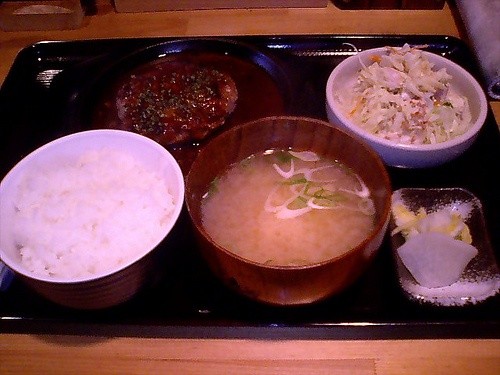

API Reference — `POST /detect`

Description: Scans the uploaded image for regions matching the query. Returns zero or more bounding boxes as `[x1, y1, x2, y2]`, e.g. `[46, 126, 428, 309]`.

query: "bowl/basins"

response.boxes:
[186, 116, 389, 304]
[0, 129, 186, 308]
[326, 48, 489, 171]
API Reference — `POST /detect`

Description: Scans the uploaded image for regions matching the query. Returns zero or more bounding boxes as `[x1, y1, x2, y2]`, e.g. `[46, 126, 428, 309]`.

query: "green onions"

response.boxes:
[263, 150, 376, 219]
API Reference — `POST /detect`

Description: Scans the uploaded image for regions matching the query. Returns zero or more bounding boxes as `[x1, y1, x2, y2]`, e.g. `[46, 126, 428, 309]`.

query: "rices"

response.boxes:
[11, 149, 176, 279]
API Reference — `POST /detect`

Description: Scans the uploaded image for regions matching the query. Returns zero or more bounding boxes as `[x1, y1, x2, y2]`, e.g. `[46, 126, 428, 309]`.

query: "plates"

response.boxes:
[90, 38, 312, 166]
[391, 186, 500, 305]
